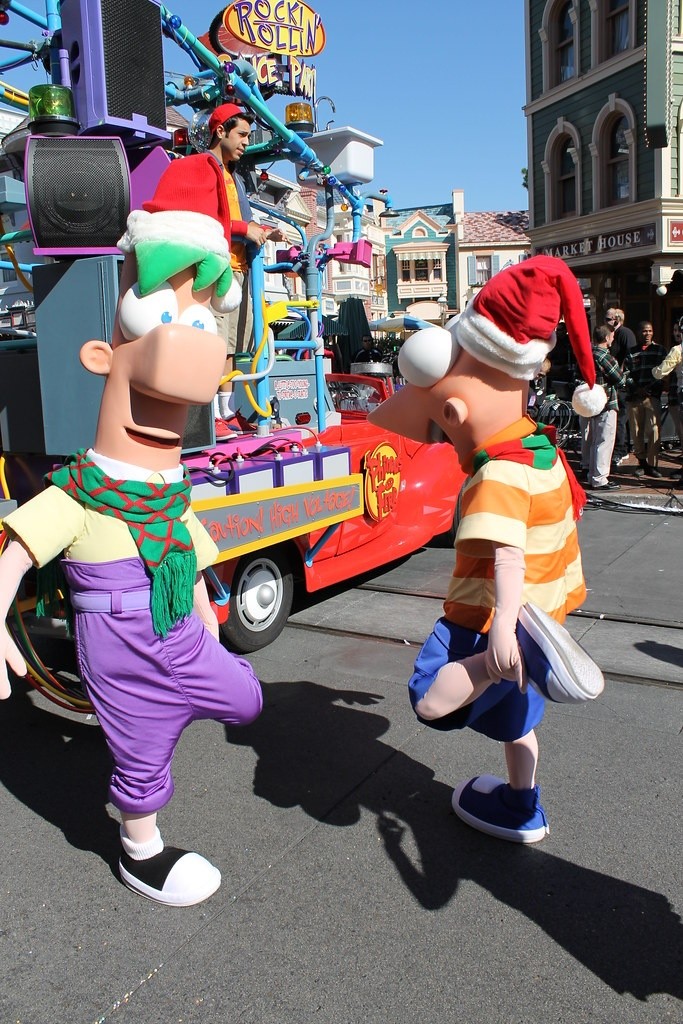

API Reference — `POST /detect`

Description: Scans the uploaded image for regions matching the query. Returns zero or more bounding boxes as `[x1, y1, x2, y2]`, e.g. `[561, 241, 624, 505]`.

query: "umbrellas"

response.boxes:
[368, 314, 442, 339]
[337, 297, 374, 374]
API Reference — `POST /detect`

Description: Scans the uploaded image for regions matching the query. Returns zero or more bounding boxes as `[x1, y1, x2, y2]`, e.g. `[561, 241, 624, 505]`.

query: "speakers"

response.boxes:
[22, 0, 216, 456]
[276, 246, 302, 264]
[334, 240, 373, 268]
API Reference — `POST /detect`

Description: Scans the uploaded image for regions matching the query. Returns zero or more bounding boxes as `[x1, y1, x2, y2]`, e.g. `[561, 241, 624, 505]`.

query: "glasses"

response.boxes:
[605, 318, 617, 322]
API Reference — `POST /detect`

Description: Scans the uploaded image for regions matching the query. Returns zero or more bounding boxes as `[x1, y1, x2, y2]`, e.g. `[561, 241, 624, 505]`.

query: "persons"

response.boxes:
[569, 323, 626, 490]
[354, 335, 383, 363]
[604, 308, 637, 465]
[623, 321, 669, 478]
[546, 322, 576, 365]
[652, 315, 683, 489]
[527, 358, 551, 421]
[204, 104, 292, 442]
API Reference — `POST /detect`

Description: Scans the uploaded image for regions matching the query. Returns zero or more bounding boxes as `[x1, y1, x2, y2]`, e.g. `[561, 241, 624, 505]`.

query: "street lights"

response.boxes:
[437, 292, 448, 327]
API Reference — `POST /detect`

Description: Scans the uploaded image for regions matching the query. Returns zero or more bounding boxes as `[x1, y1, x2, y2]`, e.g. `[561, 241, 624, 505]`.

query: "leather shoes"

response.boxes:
[594, 481, 621, 490]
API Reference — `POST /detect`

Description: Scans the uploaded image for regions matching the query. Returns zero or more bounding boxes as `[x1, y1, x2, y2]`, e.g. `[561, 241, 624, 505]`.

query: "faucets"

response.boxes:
[314, 96, 336, 133]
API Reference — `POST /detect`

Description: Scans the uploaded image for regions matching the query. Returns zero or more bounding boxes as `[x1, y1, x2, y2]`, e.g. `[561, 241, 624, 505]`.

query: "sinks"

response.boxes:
[295, 126, 383, 190]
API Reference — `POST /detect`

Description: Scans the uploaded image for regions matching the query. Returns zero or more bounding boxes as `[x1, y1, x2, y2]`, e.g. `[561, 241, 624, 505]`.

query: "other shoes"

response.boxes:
[612, 457, 622, 465]
[118, 826, 223, 907]
[671, 469, 683, 479]
[515, 602, 604, 705]
[622, 452, 631, 460]
[450, 775, 549, 843]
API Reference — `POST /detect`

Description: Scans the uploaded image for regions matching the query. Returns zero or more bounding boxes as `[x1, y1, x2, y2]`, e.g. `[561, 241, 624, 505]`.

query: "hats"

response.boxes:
[209, 104, 242, 137]
[456, 254, 608, 418]
[115, 154, 232, 258]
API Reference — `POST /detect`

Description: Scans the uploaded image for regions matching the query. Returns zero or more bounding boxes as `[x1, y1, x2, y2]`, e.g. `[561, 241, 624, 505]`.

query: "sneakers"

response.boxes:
[634, 466, 646, 476]
[645, 467, 663, 478]
[225, 408, 258, 435]
[215, 417, 237, 441]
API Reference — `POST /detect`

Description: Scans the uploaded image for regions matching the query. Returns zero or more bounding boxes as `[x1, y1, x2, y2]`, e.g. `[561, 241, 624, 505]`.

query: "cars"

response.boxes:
[0, 371, 471, 651]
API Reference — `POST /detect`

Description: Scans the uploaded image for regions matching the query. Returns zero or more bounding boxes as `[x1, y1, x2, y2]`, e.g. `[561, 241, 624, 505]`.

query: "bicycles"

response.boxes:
[534, 371, 586, 457]
[373, 335, 410, 377]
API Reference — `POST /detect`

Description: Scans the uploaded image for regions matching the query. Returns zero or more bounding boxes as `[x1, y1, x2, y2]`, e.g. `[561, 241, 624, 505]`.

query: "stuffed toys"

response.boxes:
[367, 254, 607, 843]
[0, 153, 263, 906]
